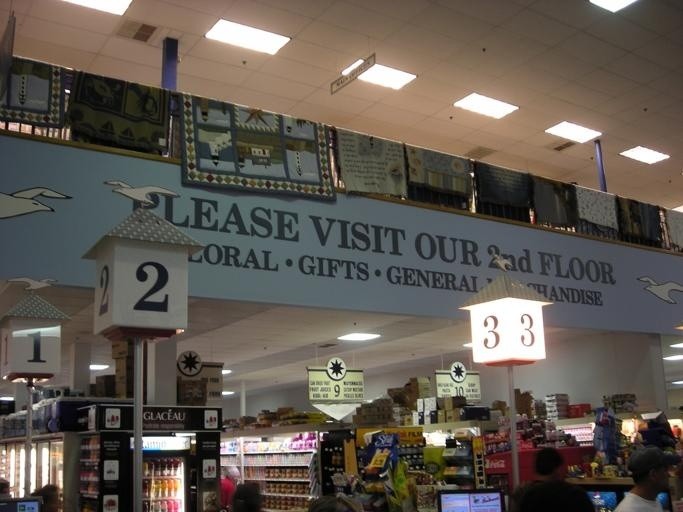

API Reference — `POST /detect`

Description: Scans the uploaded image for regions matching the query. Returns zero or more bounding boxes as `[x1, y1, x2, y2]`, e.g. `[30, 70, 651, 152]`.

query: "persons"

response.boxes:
[231, 482, 267, 511]
[0, 477, 59, 512]
[219, 466, 240, 511]
[613, 447, 682, 512]
[507, 447, 596, 512]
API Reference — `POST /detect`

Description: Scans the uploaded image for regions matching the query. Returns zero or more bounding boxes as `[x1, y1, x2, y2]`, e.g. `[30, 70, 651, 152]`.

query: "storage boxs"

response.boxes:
[514, 387, 591, 420]
[237, 405, 326, 428]
[353, 375, 511, 426]
[89, 338, 133, 398]
[176, 372, 207, 406]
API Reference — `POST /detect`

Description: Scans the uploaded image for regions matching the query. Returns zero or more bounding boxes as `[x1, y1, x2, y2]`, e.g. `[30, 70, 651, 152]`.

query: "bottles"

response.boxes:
[142, 458, 182, 512]
[79, 436, 99, 493]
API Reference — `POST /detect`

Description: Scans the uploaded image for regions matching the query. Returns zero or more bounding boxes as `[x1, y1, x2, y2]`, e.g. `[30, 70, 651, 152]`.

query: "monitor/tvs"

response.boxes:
[624, 491, 673, 512]
[16, 500, 39, 512]
[437, 488, 506, 512]
[586, 491, 617, 512]
[640, 411, 673, 437]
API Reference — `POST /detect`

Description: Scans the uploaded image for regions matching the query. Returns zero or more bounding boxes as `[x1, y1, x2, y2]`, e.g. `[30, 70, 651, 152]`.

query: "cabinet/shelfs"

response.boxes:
[219, 419, 596, 511]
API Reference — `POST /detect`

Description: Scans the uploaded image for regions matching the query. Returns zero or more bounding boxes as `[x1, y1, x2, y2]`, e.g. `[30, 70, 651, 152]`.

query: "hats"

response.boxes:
[627, 444, 682, 472]
